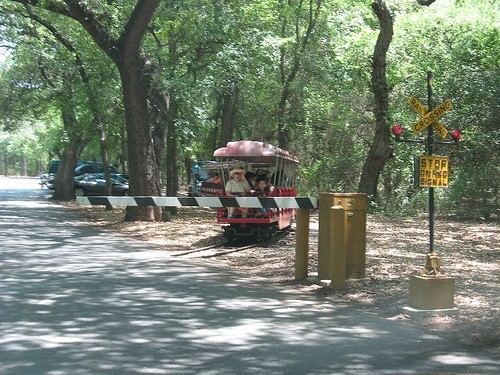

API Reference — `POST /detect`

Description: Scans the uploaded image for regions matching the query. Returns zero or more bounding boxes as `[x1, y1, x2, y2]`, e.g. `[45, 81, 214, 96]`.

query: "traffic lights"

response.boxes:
[391, 125, 402, 136]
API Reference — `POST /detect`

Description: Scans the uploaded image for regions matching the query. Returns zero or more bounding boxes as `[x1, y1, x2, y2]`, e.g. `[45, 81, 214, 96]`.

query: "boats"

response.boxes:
[448, 127, 462, 142]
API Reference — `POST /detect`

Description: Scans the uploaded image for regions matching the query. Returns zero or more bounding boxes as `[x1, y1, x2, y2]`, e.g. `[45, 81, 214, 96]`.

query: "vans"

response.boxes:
[46, 159, 121, 191]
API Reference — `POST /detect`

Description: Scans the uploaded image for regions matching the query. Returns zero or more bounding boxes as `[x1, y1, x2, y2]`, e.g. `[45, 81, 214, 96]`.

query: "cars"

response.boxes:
[69, 172, 129, 195]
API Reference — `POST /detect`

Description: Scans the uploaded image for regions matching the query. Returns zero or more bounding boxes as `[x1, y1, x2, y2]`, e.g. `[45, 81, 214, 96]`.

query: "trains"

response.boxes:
[189, 140, 307, 245]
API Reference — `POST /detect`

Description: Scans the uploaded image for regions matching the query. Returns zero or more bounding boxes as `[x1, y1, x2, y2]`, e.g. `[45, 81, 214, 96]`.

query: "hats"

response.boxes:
[229, 167, 244, 177]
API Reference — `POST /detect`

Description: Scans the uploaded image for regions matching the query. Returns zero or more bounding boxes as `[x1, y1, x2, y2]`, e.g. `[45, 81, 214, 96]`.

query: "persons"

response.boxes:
[224, 168, 252, 219]
[244, 165, 296, 192]
[207, 173, 222, 184]
[251, 177, 271, 219]
[39, 171, 49, 189]
